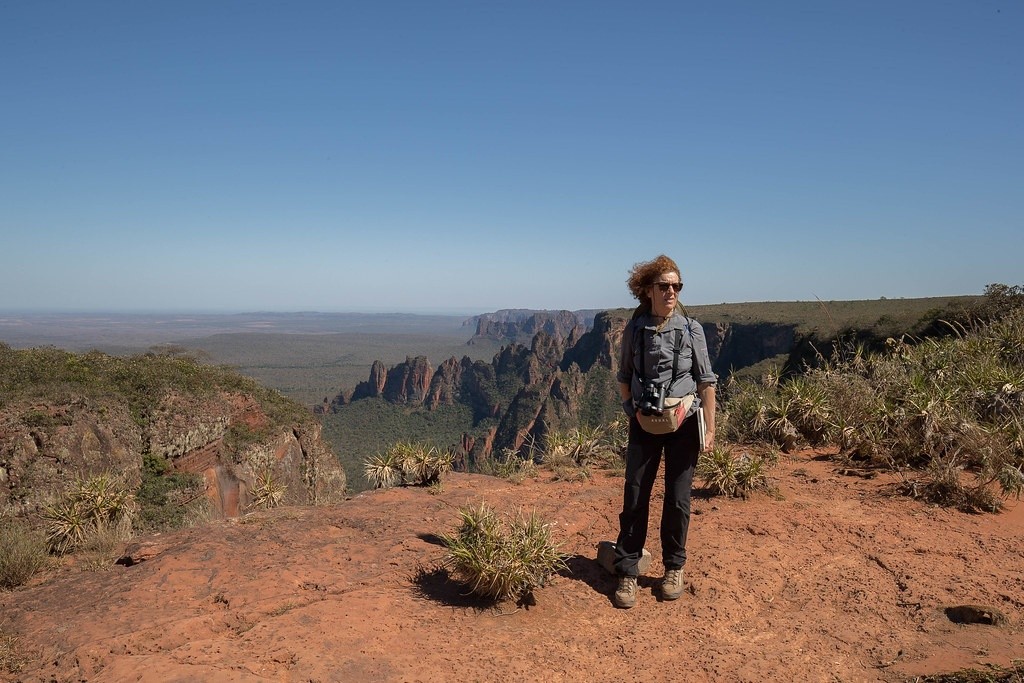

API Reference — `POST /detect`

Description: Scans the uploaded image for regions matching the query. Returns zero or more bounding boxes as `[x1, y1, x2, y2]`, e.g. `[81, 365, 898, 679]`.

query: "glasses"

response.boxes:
[648, 282, 683, 293]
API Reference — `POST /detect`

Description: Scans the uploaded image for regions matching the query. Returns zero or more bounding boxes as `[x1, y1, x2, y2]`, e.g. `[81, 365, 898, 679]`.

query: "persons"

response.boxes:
[614, 254, 717, 608]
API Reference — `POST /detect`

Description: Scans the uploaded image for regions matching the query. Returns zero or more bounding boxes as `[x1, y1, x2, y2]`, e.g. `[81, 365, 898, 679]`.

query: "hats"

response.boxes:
[632, 300, 689, 317]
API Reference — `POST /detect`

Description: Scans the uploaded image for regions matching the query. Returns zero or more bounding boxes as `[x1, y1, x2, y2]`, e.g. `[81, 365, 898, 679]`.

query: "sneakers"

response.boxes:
[661, 568, 684, 598]
[615, 577, 637, 607]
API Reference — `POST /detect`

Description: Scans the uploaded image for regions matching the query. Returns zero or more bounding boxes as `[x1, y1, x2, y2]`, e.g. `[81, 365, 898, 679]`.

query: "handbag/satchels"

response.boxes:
[632, 395, 694, 434]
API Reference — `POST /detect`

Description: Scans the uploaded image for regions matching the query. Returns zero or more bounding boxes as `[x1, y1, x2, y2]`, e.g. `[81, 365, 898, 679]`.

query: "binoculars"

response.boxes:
[640, 381, 667, 417]
